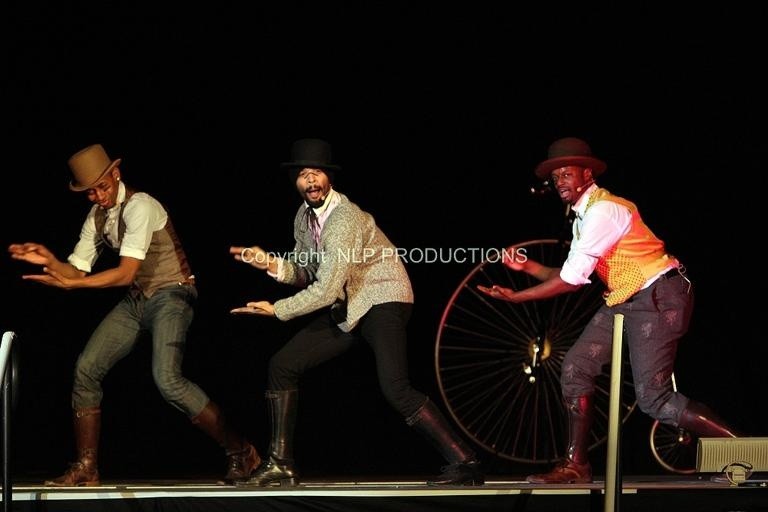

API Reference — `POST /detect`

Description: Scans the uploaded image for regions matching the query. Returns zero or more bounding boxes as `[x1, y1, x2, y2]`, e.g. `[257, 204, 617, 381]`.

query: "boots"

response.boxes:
[187, 396, 262, 486]
[678, 398, 754, 484]
[43, 405, 102, 488]
[526, 389, 595, 485]
[403, 395, 486, 487]
[233, 386, 301, 489]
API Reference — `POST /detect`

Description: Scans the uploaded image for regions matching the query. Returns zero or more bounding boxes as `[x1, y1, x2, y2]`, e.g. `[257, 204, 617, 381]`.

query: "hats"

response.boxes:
[66, 143, 123, 193]
[534, 137, 609, 180]
[280, 138, 343, 172]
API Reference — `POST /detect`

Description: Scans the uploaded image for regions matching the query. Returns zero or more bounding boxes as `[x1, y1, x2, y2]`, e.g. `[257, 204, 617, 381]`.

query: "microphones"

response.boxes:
[99, 206, 104, 210]
[319, 191, 331, 200]
[577, 181, 596, 193]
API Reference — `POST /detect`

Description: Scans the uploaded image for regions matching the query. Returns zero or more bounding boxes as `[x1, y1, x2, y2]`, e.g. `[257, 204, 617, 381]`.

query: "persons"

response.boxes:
[475, 135, 758, 485]
[229, 136, 487, 488]
[6, 141, 265, 487]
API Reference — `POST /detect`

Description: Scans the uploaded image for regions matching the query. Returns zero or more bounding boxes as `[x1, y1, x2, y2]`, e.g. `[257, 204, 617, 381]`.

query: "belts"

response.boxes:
[665, 268, 687, 278]
